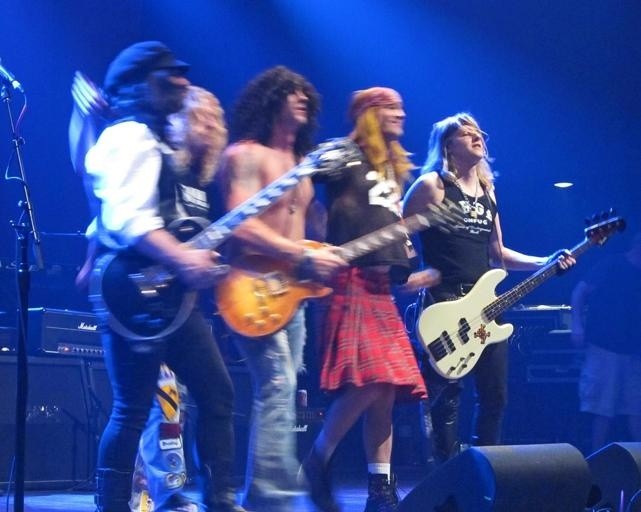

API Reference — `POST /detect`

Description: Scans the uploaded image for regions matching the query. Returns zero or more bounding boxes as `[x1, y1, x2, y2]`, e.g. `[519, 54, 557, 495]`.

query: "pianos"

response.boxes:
[501, 306, 574, 331]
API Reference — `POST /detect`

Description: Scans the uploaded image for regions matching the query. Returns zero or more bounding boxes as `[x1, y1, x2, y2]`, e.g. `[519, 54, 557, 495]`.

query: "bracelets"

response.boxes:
[293, 243, 316, 276]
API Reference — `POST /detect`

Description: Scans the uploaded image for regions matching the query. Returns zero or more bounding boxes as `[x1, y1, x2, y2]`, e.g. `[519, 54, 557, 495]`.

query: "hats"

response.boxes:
[104, 40, 192, 91]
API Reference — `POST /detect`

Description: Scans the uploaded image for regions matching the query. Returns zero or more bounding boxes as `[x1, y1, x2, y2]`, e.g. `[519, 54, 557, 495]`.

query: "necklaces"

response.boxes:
[276, 153, 302, 218]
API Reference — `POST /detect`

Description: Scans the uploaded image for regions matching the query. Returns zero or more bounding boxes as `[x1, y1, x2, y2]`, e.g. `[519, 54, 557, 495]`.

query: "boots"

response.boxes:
[363, 471, 402, 512]
[299, 446, 338, 511]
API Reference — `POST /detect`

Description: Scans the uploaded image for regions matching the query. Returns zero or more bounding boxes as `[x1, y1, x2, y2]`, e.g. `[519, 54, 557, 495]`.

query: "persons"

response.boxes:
[400, 110, 577, 476]
[65, 70, 231, 511]
[565, 233, 641, 455]
[78, 39, 249, 509]
[292, 83, 449, 511]
[214, 66, 356, 508]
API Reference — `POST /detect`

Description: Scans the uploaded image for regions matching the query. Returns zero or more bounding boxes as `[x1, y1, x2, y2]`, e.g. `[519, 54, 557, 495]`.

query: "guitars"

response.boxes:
[90, 139, 361, 338]
[214, 200, 464, 338]
[415, 208, 627, 380]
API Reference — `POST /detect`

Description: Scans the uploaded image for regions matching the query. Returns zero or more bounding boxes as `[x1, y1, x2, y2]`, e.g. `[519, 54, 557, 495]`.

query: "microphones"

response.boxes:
[0, 65, 25, 94]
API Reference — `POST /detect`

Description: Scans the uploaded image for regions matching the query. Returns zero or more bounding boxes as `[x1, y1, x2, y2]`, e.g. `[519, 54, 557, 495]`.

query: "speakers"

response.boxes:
[584, 442, 641, 512]
[393, 443, 593, 512]
[0, 355, 93, 490]
[86, 360, 114, 490]
